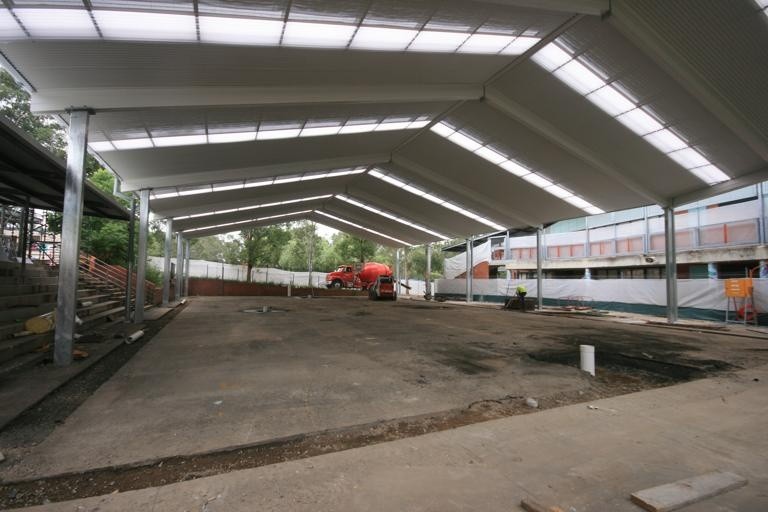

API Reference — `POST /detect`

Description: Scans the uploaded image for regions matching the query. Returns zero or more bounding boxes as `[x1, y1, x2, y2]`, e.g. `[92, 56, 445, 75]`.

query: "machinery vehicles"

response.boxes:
[325, 262, 393, 291]
[368, 274, 396, 301]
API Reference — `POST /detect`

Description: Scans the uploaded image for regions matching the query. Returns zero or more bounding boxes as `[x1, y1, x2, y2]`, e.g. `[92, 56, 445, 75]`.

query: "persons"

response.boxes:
[516, 286, 527, 313]
